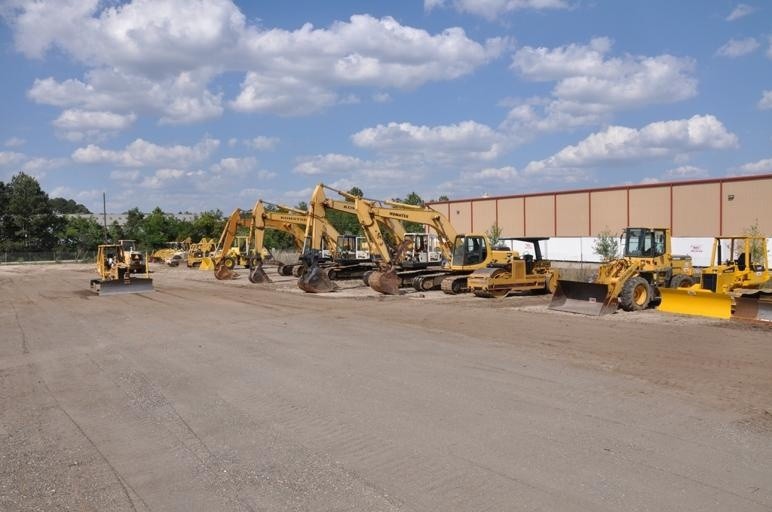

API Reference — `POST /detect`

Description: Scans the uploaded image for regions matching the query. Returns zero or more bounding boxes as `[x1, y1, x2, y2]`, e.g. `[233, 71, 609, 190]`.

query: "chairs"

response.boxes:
[737, 253, 751, 270]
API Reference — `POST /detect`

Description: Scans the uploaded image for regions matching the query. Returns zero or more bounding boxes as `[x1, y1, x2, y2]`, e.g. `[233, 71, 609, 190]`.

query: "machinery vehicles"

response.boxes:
[87, 239, 155, 297]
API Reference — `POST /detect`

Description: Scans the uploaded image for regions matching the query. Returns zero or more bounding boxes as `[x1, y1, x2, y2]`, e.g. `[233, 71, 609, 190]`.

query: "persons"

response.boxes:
[211, 244, 216, 251]
[646, 242, 657, 254]
